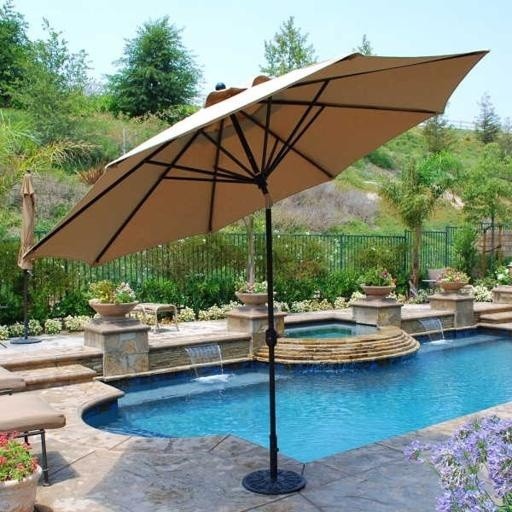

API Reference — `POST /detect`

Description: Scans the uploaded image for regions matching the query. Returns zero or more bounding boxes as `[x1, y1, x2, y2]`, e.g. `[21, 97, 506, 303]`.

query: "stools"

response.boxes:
[1, 394, 66, 486]
[0, 364, 29, 393]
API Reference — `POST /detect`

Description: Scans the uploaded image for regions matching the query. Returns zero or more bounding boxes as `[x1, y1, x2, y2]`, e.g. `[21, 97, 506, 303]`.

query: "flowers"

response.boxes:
[356, 265, 398, 286]
[87, 278, 136, 305]
[435, 268, 469, 282]
[0, 429, 40, 482]
[233, 280, 271, 294]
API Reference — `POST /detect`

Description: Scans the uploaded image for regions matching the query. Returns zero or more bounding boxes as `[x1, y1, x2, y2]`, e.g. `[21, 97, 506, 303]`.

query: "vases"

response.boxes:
[360, 284, 396, 298]
[88, 299, 139, 317]
[0, 464, 43, 512]
[235, 291, 276, 306]
[437, 283, 467, 293]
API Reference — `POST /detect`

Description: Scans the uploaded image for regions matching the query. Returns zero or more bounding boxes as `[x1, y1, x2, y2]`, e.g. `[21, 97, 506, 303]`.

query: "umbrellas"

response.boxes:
[18, 48, 492, 483]
[17, 165, 40, 339]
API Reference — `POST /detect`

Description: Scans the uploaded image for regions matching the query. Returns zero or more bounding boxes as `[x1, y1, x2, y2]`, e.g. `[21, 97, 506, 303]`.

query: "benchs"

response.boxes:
[127, 303, 181, 334]
